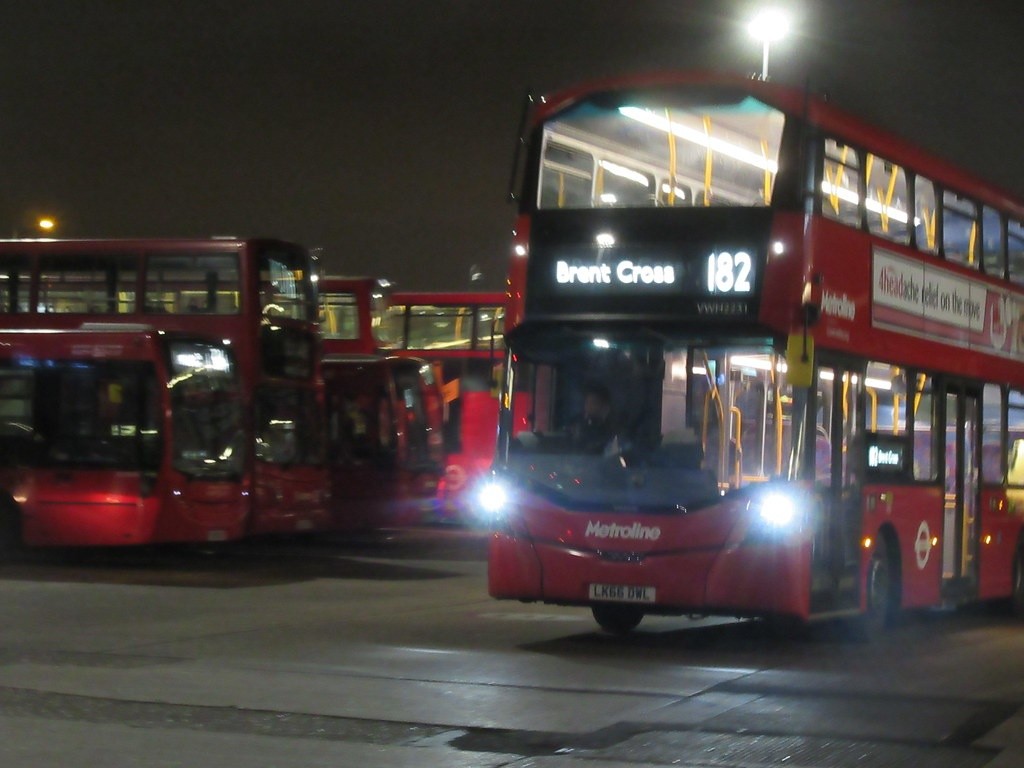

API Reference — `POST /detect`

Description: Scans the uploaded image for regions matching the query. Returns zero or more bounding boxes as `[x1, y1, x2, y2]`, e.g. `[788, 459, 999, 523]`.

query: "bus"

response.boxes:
[349, 288, 508, 551]
[480, 69, 1024, 637]
[0, 321, 256, 573]
[0, 234, 328, 542]
[232, 275, 447, 545]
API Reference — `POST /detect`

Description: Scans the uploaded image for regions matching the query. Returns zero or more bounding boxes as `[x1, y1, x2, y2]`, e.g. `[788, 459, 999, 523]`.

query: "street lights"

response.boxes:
[732, 4, 793, 81]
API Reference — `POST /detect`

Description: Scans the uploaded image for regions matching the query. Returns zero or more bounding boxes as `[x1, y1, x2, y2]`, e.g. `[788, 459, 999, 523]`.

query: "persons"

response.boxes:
[566, 382, 632, 456]
